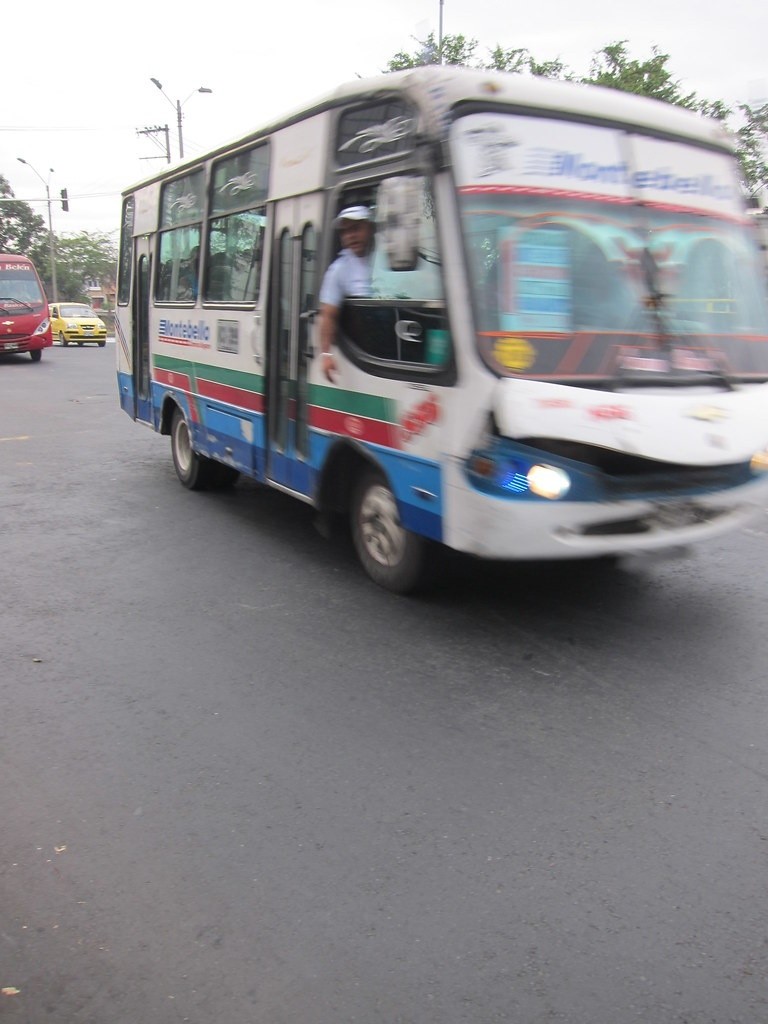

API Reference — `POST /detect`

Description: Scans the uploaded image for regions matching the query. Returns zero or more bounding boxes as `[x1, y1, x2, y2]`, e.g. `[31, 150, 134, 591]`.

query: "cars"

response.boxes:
[47, 301, 107, 347]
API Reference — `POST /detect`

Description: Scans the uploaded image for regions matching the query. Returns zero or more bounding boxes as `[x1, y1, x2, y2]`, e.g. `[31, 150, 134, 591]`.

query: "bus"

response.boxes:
[0, 252, 53, 362]
[112, 62, 768, 596]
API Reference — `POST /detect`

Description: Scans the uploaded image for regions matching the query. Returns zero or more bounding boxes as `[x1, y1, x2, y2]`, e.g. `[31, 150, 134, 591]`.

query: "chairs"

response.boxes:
[151, 246, 261, 299]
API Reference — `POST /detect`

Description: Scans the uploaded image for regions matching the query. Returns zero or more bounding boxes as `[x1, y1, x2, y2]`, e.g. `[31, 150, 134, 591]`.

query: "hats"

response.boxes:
[331, 205, 372, 228]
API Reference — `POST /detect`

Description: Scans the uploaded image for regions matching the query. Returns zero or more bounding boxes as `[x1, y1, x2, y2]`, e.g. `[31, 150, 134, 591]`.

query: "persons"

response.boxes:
[8, 282, 36, 302]
[318, 206, 405, 384]
[179, 246, 211, 299]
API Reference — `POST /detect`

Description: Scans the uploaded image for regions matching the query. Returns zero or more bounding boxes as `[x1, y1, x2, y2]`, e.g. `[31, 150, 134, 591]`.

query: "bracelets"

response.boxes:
[320, 353, 333, 357]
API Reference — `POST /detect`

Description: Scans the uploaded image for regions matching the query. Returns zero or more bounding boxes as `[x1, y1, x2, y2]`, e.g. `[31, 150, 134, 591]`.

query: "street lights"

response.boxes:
[16, 156, 59, 303]
[150, 77, 212, 159]
